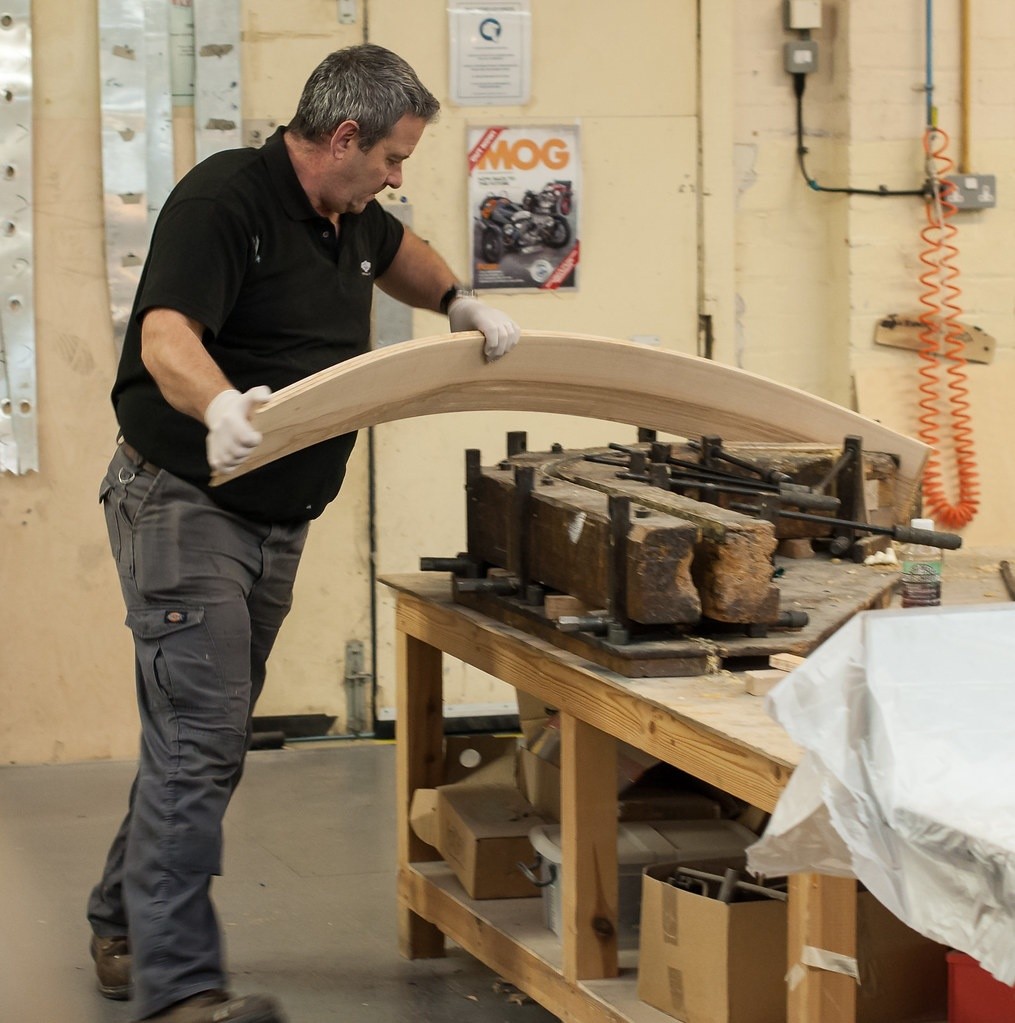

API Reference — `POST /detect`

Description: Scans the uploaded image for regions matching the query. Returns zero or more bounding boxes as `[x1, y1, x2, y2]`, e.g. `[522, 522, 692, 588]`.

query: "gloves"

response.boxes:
[201, 386, 270, 477]
[448, 296, 520, 361]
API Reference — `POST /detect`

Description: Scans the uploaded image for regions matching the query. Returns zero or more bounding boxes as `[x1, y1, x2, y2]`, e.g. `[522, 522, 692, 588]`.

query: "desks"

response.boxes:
[366, 566, 955, 1023]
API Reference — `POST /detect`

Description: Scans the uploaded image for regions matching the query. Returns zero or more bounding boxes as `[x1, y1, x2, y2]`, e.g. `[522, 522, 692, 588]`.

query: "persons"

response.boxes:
[86, 42, 522, 1023]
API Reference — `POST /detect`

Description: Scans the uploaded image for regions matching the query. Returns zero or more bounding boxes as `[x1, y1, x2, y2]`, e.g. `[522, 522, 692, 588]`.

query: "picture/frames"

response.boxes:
[466, 121, 582, 296]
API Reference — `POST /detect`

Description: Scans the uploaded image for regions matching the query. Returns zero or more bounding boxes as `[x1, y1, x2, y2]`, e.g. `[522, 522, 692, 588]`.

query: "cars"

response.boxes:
[479, 181, 574, 264]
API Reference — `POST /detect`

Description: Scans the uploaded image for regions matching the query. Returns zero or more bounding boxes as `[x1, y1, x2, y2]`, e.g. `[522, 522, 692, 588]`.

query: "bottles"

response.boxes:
[902, 517, 942, 607]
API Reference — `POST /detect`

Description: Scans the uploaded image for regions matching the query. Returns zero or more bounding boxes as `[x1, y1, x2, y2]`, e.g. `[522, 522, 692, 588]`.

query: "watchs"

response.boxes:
[440, 282, 478, 315]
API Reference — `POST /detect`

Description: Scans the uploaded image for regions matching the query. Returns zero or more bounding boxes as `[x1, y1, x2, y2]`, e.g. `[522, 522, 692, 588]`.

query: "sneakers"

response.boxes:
[141, 992, 287, 1023]
[86, 931, 134, 999]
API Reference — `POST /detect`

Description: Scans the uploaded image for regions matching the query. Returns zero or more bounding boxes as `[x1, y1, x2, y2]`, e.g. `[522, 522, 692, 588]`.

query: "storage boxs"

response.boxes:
[782, 864, 949, 1022]
[414, 730, 720, 900]
[945, 950, 1015, 1023]
[634, 857, 788, 1023]
